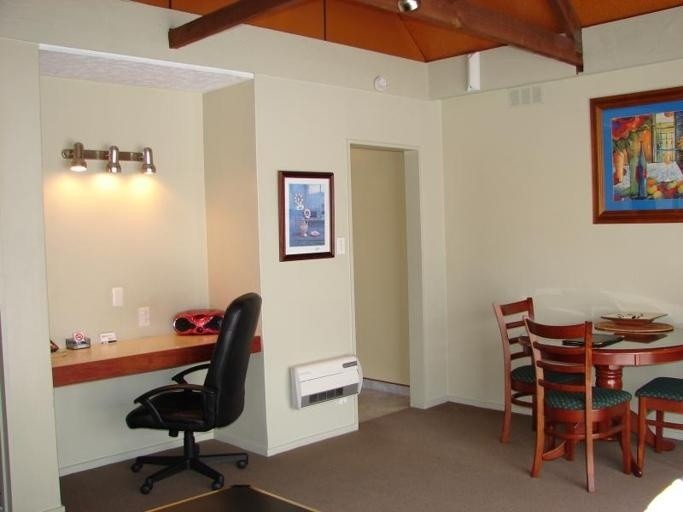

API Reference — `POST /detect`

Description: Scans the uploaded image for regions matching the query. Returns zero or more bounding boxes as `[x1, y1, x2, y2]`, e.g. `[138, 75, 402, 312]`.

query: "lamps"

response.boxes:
[61, 142, 156, 176]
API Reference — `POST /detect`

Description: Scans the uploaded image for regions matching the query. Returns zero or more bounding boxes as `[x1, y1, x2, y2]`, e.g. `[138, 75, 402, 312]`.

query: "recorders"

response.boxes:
[172, 308, 224, 336]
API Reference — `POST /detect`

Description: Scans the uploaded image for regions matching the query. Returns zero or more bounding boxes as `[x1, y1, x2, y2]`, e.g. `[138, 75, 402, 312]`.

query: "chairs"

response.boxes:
[492, 297, 682, 493]
[125, 292, 262, 495]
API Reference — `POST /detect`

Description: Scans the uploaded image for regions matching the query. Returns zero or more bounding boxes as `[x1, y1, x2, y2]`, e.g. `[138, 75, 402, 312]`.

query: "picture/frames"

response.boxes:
[277, 170, 335, 262]
[589, 85, 683, 223]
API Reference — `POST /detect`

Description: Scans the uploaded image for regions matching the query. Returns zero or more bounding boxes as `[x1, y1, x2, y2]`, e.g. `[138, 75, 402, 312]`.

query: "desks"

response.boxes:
[51, 332, 262, 388]
